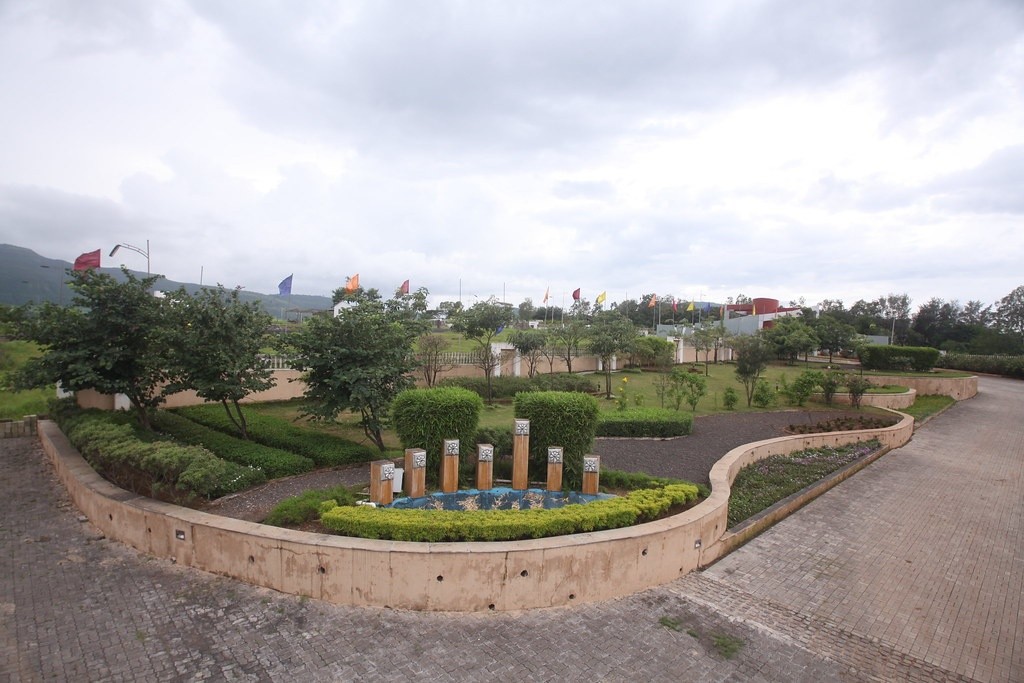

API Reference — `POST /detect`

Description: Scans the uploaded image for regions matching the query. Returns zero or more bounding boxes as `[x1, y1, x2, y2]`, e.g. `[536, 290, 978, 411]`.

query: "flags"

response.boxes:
[598, 292, 606, 303]
[673, 303, 677, 311]
[278, 274, 292, 297]
[400, 280, 409, 293]
[346, 274, 359, 295]
[705, 303, 712, 312]
[543, 287, 549, 302]
[74, 249, 100, 272]
[648, 294, 656, 307]
[687, 300, 695, 311]
[573, 288, 580, 299]
